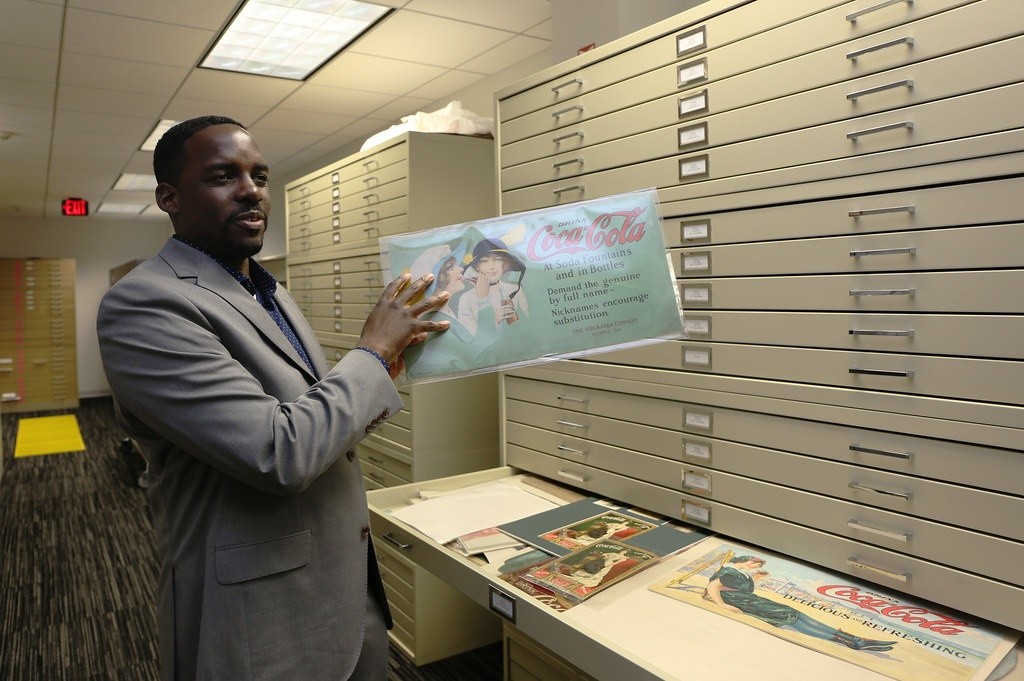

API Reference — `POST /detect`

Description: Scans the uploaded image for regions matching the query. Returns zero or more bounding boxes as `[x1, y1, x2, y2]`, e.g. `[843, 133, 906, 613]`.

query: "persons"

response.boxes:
[96, 116, 450, 681]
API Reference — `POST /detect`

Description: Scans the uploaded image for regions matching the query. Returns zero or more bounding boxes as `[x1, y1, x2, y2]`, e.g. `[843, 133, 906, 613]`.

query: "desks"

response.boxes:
[365, 464, 1024, 681]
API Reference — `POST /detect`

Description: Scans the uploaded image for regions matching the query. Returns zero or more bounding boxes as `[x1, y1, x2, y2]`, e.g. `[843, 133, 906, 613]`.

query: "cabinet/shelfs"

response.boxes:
[0, 257, 79, 412]
[370, 534, 501, 666]
[503, 618, 598, 680]
[283, 128, 500, 492]
[494, 0, 1023, 636]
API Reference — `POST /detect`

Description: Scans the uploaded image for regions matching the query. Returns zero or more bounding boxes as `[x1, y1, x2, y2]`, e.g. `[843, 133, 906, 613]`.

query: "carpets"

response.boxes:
[14, 413, 85, 457]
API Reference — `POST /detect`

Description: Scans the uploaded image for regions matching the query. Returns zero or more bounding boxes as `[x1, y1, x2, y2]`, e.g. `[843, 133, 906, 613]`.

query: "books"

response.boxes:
[409, 480, 659, 613]
